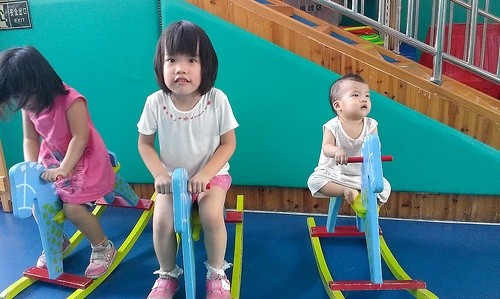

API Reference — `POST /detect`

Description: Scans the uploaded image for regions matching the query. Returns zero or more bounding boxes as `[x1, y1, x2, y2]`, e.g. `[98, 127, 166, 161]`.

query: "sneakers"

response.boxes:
[85, 239, 116, 278]
[36, 234, 72, 269]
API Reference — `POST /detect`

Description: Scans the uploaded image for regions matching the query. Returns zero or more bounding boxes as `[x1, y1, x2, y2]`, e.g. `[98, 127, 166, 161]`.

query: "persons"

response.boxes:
[307, 72, 392, 209]
[136, 19, 240, 299]
[0, 45, 117, 280]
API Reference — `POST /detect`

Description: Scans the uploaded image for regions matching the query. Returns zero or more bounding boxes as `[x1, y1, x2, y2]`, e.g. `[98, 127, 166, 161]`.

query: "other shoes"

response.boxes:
[147, 276, 179, 299]
[206, 273, 231, 299]
